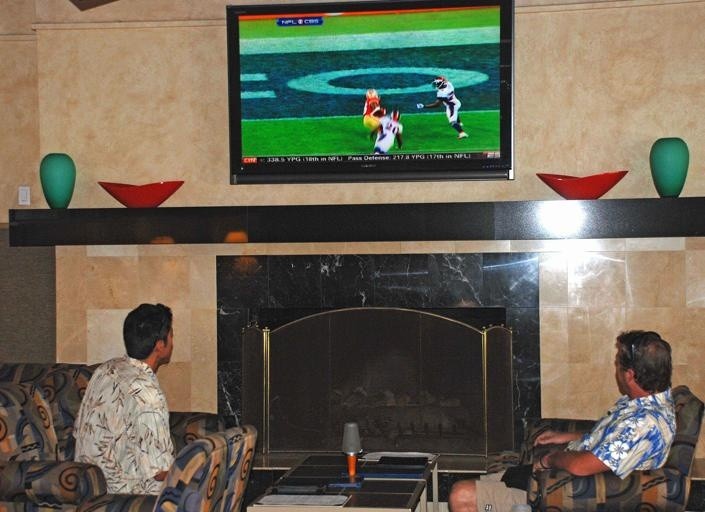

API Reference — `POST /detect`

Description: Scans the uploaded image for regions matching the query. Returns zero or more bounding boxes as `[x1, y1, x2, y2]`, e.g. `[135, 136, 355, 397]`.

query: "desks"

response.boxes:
[243, 452, 439, 512]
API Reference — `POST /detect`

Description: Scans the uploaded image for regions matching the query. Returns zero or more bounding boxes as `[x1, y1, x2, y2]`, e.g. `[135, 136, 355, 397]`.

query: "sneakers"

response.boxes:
[460, 123, 464, 130]
[458, 132, 468, 139]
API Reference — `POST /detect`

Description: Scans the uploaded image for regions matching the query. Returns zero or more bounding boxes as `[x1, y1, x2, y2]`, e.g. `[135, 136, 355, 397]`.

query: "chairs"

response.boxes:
[1, 361, 258, 512]
[487, 386, 705, 512]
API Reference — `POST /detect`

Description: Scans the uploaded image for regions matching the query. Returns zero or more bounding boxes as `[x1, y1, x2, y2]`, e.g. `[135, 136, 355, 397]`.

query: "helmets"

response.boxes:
[390, 109, 401, 121]
[365, 88, 378, 99]
[432, 77, 448, 89]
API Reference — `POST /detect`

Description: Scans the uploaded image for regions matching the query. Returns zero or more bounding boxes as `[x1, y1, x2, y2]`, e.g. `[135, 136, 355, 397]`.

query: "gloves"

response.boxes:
[417, 103, 424, 109]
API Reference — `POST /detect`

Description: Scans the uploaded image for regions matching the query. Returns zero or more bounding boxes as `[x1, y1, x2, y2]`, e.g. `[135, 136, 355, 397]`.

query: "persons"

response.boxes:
[448, 331, 677, 512]
[72, 303, 175, 495]
[363, 89, 403, 155]
[417, 77, 469, 139]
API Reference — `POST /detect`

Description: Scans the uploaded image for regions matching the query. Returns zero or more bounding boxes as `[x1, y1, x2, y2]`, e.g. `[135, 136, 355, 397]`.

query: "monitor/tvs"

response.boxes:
[226, 0, 515, 185]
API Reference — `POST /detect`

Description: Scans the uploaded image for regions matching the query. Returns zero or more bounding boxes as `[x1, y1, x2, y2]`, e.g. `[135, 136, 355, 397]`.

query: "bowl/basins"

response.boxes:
[96, 181, 187, 208]
[535, 171, 629, 200]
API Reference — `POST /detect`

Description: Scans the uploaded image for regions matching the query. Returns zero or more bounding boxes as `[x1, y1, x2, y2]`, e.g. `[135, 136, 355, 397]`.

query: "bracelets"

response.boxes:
[540, 453, 552, 469]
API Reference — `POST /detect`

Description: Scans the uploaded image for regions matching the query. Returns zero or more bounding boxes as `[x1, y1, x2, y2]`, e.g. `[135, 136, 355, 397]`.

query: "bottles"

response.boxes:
[39, 153, 76, 209]
[650, 138, 689, 198]
